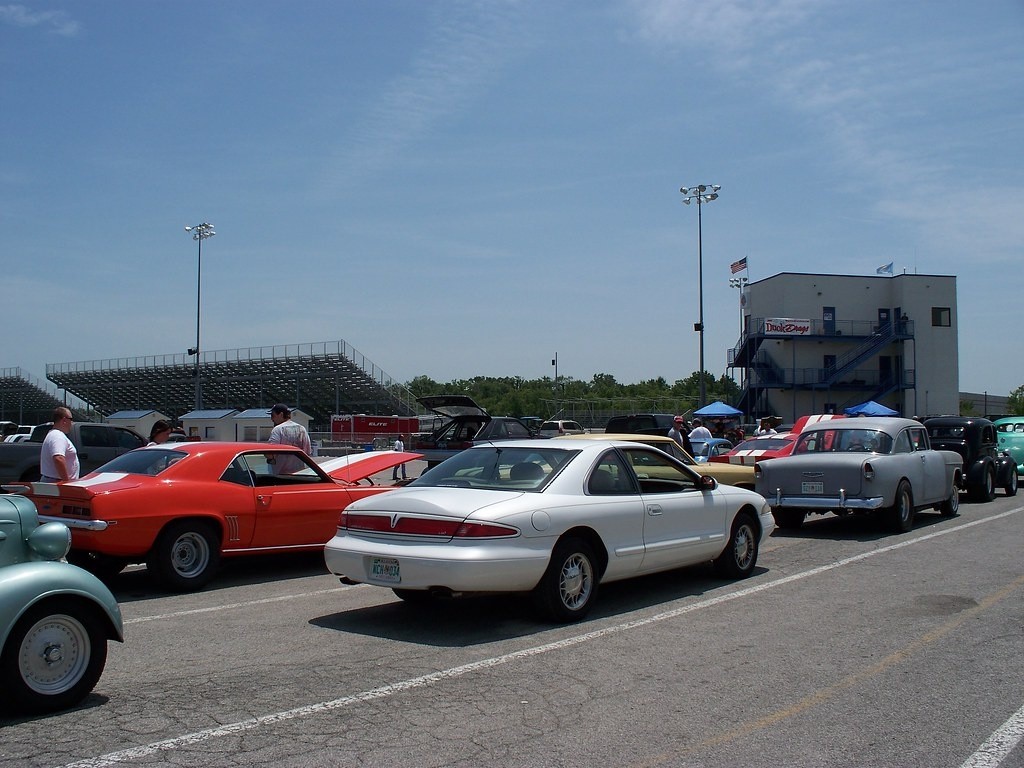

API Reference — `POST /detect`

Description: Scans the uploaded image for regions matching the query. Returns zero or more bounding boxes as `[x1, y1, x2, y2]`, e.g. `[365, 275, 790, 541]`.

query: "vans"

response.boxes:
[605, 414, 694, 456]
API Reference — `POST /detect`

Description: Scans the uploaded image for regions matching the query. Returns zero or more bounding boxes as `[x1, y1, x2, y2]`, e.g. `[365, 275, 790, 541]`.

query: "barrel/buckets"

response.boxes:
[836, 331, 841, 336]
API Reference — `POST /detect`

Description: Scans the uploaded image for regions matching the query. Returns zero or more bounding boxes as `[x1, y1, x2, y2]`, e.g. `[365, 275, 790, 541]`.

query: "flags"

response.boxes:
[730, 256, 747, 274]
[876, 261, 893, 274]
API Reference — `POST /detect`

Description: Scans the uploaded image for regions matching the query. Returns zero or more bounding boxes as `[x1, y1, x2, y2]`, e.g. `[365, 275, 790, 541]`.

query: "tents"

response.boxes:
[841, 401, 899, 417]
[692, 401, 745, 427]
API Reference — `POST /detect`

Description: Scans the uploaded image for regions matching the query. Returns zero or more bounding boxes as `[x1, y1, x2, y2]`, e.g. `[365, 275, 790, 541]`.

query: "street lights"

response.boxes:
[681, 182, 724, 409]
[185, 221, 216, 410]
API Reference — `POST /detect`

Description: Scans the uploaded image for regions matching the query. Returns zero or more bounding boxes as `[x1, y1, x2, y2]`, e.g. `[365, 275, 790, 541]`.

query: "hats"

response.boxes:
[673, 415, 683, 423]
[266, 403, 291, 414]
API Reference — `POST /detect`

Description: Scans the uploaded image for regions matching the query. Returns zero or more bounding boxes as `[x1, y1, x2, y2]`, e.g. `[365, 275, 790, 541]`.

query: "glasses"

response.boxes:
[61, 417, 74, 421]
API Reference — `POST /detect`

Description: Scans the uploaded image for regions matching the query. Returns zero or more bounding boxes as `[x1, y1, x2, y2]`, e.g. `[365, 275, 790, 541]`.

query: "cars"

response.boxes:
[0, 420, 35, 444]
[411, 393, 538, 469]
[25, 441, 425, 596]
[705, 412, 847, 467]
[454, 431, 758, 494]
[755, 416, 967, 532]
[688, 436, 734, 464]
[988, 416, 1024, 477]
[535, 419, 586, 438]
[914, 415, 1018, 502]
[0, 492, 128, 716]
[324, 437, 777, 625]
[772, 424, 793, 433]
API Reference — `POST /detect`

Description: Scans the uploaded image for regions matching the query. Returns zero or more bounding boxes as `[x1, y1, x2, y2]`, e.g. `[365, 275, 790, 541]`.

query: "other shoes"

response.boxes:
[402, 477, 410, 480]
[393, 478, 401, 480]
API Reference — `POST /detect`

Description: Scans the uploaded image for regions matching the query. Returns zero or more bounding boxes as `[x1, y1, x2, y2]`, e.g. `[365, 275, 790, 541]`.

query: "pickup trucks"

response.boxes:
[0, 423, 150, 495]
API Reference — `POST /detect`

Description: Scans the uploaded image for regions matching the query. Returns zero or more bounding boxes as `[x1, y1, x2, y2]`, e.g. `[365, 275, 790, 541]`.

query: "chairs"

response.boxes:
[509, 462, 545, 480]
[589, 469, 620, 491]
[468, 425, 477, 437]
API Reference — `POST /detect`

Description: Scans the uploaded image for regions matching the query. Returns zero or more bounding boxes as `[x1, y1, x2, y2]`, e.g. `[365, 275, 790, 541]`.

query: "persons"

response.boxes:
[753, 422, 777, 437]
[910, 415, 920, 442]
[668, 416, 744, 460]
[39, 407, 79, 483]
[847, 414, 875, 450]
[392, 434, 410, 481]
[262, 404, 312, 476]
[146, 421, 186, 447]
